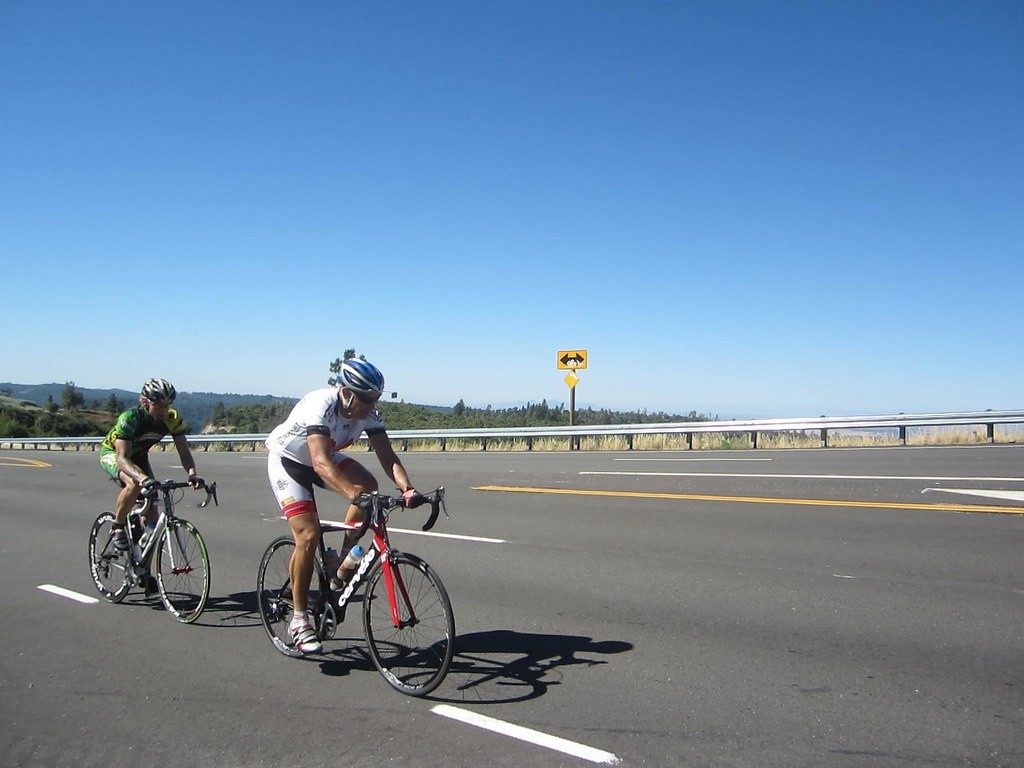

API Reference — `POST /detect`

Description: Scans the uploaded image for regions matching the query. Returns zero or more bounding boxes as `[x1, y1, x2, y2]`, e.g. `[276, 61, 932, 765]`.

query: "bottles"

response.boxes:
[326, 547, 342, 591]
[138, 521, 156, 549]
[336, 545, 366, 582]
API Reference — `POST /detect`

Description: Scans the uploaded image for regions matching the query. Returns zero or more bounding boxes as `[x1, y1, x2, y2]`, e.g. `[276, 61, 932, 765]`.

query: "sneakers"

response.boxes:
[337, 564, 370, 581]
[288, 620, 323, 654]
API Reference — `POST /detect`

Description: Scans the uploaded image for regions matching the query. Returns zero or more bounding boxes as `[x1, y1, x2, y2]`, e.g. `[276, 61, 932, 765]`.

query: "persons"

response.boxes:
[266, 357, 422, 654]
[101, 378, 205, 586]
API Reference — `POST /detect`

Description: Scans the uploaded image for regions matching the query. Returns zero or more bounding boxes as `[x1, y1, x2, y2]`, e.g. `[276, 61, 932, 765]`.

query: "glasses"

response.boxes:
[147, 399, 173, 409]
[348, 388, 381, 404]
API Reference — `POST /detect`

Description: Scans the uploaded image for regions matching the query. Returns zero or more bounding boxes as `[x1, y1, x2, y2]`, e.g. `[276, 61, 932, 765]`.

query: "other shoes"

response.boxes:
[109, 526, 129, 551]
[139, 576, 159, 593]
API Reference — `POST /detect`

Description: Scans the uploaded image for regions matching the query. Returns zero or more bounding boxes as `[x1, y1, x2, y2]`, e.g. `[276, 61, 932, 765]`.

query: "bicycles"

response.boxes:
[88, 477, 220, 625]
[256, 483, 457, 697]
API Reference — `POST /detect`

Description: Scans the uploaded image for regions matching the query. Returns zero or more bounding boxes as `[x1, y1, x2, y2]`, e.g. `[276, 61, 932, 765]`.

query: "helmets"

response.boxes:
[340, 358, 384, 394]
[141, 378, 176, 404]
